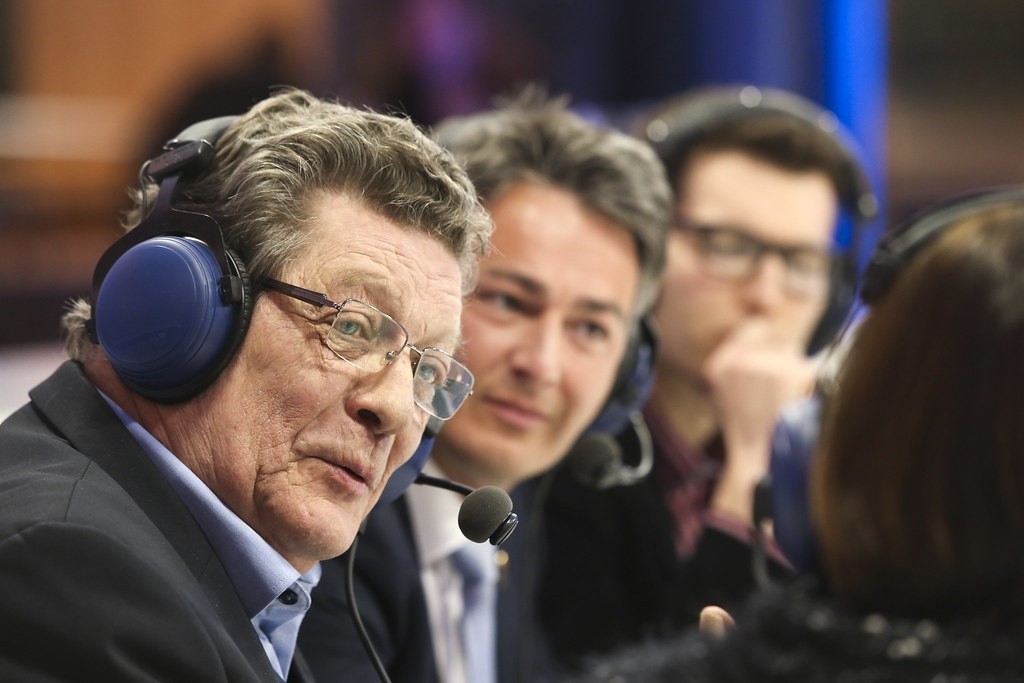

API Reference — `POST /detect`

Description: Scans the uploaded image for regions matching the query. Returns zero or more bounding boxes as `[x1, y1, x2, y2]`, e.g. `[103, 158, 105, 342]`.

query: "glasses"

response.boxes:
[256, 275, 475, 421]
[673, 225, 846, 290]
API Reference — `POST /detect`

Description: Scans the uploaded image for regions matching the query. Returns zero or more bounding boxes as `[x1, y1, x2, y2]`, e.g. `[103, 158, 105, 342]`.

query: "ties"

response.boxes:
[448, 536, 501, 683]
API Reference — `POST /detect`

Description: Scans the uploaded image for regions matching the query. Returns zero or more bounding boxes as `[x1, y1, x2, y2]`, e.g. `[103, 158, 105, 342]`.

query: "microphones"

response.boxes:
[414, 472, 519, 546]
[581, 410, 653, 488]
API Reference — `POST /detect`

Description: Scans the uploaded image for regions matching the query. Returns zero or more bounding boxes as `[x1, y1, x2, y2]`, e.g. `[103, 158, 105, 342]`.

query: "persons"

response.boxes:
[575, 86, 876, 609]
[0, 91, 492, 683]
[347, 104, 676, 683]
[609, 203, 1024, 683]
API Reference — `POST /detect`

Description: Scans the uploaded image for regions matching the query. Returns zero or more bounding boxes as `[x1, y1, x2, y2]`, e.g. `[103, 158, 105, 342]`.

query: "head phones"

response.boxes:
[86, 116, 443, 505]
[564, 77, 1024, 577]
